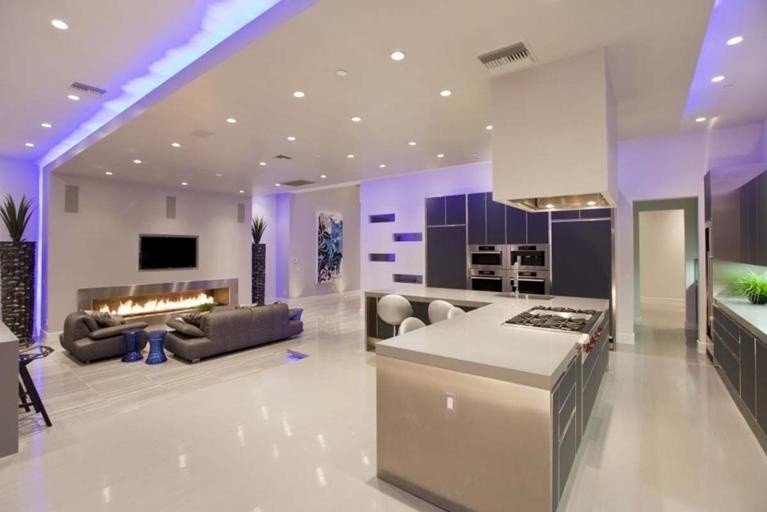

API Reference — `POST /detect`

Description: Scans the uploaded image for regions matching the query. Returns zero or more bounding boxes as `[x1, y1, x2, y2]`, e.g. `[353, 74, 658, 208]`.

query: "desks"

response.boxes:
[0, 318, 22, 458]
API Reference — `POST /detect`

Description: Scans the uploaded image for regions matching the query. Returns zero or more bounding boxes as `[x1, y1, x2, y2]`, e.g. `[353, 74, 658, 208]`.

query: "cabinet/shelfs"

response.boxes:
[738, 170, 767, 268]
[712, 303, 767, 456]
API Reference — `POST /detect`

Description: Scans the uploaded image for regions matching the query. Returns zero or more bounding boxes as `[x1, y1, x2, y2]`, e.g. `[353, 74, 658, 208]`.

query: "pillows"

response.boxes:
[181, 314, 202, 328]
[272, 300, 297, 320]
[84, 309, 120, 326]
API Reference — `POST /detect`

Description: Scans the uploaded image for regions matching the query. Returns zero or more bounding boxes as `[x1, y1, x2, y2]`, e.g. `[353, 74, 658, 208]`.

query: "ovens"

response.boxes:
[466, 243, 552, 295]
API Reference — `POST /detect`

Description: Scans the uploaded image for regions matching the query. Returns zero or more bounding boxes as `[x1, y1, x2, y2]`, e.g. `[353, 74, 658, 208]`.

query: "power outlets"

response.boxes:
[445, 392, 456, 415]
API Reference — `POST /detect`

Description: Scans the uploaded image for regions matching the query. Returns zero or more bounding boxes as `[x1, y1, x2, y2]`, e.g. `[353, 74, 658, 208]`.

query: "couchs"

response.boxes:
[163, 303, 304, 363]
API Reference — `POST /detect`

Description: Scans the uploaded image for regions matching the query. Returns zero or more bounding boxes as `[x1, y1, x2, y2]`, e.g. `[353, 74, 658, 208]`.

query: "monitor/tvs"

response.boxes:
[138, 233, 198, 269]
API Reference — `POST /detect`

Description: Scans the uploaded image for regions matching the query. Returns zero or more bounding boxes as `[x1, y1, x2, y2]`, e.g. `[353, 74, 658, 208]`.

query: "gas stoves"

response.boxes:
[500, 304, 606, 353]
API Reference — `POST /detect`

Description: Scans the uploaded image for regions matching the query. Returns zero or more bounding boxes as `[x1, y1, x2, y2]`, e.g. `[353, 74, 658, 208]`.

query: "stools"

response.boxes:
[17, 337, 55, 426]
[145, 330, 168, 365]
[120, 329, 144, 362]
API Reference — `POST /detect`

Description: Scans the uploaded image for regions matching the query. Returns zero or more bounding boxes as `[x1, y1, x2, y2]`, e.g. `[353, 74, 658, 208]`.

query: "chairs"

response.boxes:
[59, 312, 149, 365]
[376, 292, 465, 338]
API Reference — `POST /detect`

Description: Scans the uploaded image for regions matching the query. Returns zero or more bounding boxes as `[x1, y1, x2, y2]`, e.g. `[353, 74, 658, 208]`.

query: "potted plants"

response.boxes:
[0, 189, 38, 344]
[196, 302, 215, 314]
[729, 269, 767, 305]
[251, 216, 268, 306]
